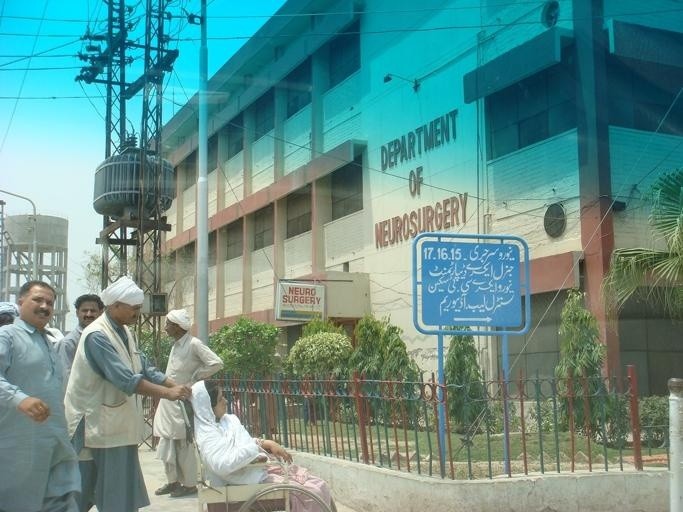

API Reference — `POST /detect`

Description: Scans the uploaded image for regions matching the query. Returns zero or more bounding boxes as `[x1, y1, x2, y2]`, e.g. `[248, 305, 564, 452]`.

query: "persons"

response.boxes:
[186, 378, 337, 512]
[53, 293, 105, 512]
[151, 308, 225, 498]
[63, 274, 194, 512]
[0, 281, 81, 512]
[0, 300, 18, 325]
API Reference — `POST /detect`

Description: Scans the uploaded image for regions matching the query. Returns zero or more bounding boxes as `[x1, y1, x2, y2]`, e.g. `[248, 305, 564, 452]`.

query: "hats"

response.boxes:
[0, 301, 20, 319]
[99, 276, 144, 307]
[166, 308, 191, 331]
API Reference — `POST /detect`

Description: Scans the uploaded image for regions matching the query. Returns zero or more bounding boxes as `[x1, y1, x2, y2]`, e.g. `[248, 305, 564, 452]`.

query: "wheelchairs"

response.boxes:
[174, 397, 331, 512]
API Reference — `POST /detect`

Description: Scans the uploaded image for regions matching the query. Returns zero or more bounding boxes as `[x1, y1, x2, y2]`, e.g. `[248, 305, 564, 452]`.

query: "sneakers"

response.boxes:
[168, 486, 198, 497]
[154, 482, 180, 495]
